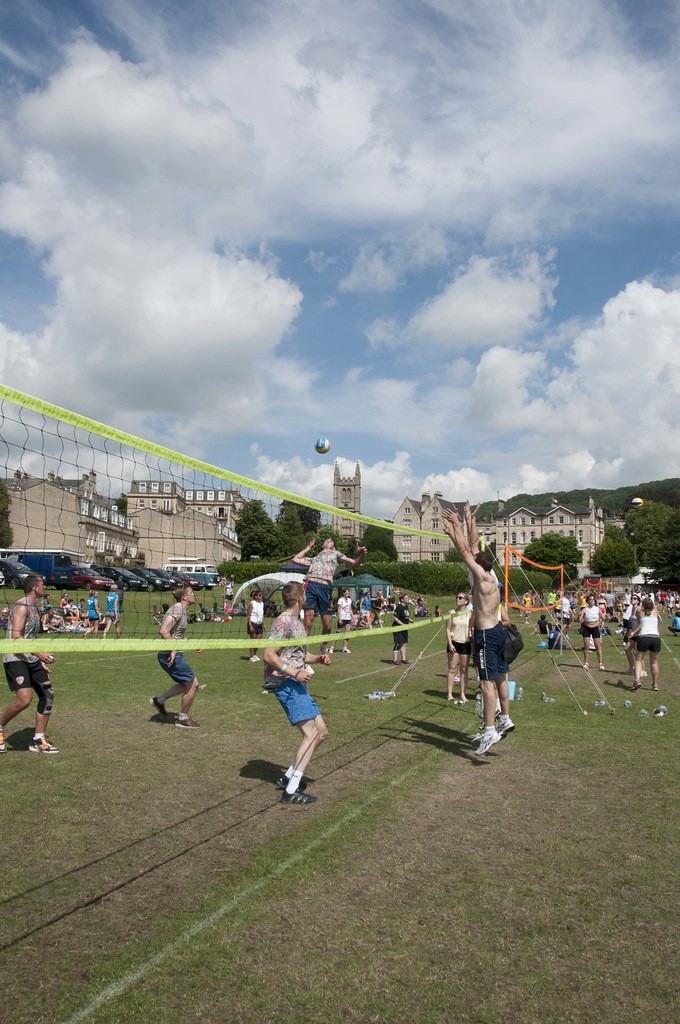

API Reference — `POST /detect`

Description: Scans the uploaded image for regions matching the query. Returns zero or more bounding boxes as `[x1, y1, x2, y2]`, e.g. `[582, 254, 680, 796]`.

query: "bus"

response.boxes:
[162, 563, 221, 590]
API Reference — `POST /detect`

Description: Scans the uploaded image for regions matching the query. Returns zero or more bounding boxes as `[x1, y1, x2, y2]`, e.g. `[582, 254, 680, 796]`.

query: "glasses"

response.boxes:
[631, 597, 638, 600]
[456, 596, 467, 600]
[566, 593, 569, 595]
[256, 594, 262, 596]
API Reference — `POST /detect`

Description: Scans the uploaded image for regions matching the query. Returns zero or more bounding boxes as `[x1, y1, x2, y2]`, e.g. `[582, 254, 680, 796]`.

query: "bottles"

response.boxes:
[475, 692, 482, 717]
[615, 626, 627, 636]
[544, 698, 554, 702]
[517, 685, 523, 700]
[363, 690, 396, 699]
[594, 699, 604, 707]
[659, 704, 667, 714]
[625, 699, 632, 709]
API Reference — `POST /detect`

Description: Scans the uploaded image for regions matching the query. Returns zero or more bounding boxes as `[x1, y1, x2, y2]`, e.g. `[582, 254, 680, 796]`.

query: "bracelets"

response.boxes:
[280, 663, 287, 673]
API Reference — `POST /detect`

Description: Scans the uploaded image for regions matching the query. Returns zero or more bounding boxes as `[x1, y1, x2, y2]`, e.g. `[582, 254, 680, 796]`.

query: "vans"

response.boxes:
[6, 553, 74, 590]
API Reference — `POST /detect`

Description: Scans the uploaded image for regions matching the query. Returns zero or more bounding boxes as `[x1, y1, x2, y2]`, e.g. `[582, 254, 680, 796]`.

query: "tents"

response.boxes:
[331, 574, 393, 600]
[232, 572, 306, 609]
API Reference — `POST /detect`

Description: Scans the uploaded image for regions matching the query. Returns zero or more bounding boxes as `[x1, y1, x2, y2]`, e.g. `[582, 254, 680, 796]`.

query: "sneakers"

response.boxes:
[0, 725, 7, 752]
[321, 644, 329, 656]
[474, 731, 502, 755]
[280, 788, 317, 804]
[29, 734, 60, 753]
[150, 697, 167, 716]
[175, 717, 200, 728]
[277, 778, 307, 791]
[496, 717, 515, 741]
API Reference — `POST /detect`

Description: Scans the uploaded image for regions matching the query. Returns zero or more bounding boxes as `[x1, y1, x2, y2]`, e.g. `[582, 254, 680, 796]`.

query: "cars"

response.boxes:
[93, 566, 148, 591]
[70, 565, 115, 591]
[0, 571, 6, 589]
[0, 559, 47, 589]
[166, 570, 201, 590]
[145, 569, 185, 589]
[127, 568, 172, 591]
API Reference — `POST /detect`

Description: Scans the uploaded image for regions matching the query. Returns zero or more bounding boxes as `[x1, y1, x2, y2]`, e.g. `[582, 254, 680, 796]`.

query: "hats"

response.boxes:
[564, 590, 572, 594]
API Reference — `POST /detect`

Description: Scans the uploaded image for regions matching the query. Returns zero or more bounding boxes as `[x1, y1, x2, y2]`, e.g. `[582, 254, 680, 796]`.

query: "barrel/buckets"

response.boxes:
[506, 681, 516, 700]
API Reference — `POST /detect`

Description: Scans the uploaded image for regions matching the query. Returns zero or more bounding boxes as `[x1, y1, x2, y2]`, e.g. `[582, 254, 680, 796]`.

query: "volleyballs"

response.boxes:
[315, 438, 330, 454]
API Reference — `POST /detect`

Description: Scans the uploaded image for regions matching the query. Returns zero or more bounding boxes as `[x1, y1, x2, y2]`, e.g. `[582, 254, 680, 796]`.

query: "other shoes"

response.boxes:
[344, 648, 351, 654]
[623, 642, 626, 646]
[329, 646, 334, 654]
[600, 662, 604, 670]
[641, 671, 647, 677]
[252, 654, 260, 661]
[630, 680, 641, 691]
[583, 663, 589, 668]
[250, 656, 258, 662]
[653, 684, 658, 692]
[402, 660, 410, 664]
[625, 668, 636, 675]
[393, 660, 401, 665]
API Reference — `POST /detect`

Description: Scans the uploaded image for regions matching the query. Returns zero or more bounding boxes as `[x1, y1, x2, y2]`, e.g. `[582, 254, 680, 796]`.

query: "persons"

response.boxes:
[149, 585, 200, 728]
[238, 599, 246, 612]
[615, 586, 680, 691]
[385, 592, 414, 664]
[408, 595, 440, 617]
[247, 590, 267, 662]
[291, 538, 367, 666]
[439, 499, 615, 756]
[265, 601, 278, 618]
[224, 579, 234, 610]
[0, 575, 59, 753]
[115, 576, 125, 613]
[261, 580, 331, 804]
[82, 590, 102, 639]
[40, 592, 88, 633]
[329, 588, 387, 654]
[102, 583, 121, 639]
